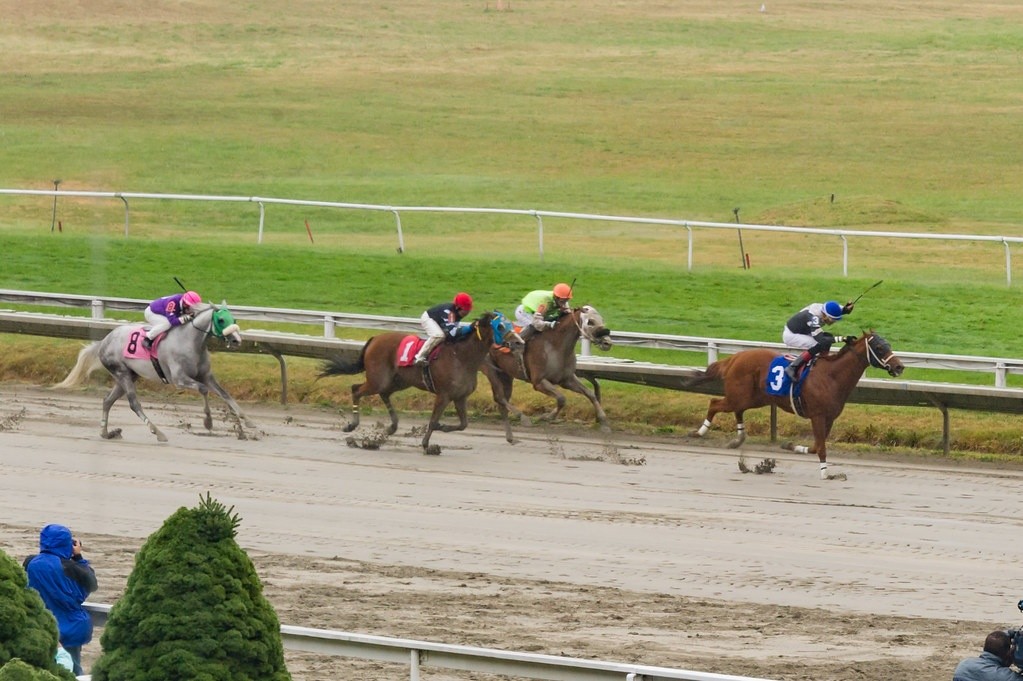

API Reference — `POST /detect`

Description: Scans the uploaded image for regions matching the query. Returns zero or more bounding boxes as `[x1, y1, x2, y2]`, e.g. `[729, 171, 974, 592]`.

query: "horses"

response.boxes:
[46, 299, 257, 441]
[312, 308, 525, 450]
[679, 327, 905, 480]
[476, 305, 613, 445]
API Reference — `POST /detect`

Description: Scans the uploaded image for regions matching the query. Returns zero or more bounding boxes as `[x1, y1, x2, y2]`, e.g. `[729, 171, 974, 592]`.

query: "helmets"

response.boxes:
[455, 293, 472, 310]
[823, 302, 842, 321]
[183, 291, 202, 307]
[555, 283, 573, 301]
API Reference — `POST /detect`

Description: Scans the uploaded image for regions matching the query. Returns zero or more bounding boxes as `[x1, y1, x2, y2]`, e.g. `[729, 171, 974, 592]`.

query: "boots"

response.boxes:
[786, 358, 802, 384]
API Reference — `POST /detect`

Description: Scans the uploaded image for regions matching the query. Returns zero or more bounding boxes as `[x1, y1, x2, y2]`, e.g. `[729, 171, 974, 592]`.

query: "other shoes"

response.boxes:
[141, 338, 152, 348]
[413, 356, 429, 367]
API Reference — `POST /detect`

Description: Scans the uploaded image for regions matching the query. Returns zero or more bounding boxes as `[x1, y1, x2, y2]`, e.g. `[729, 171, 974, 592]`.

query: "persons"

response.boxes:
[141, 291, 202, 350]
[783, 301, 854, 382]
[413, 292, 472, 364]
[511, 283, 573, 343]
[953, 631, 1023, 681]
[21, 523, 98, 675]
